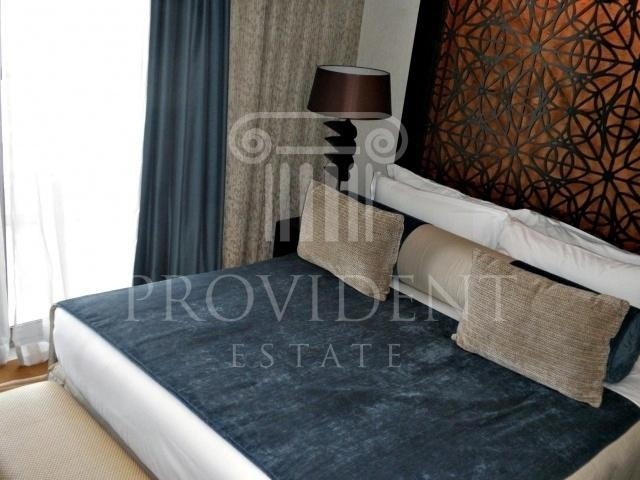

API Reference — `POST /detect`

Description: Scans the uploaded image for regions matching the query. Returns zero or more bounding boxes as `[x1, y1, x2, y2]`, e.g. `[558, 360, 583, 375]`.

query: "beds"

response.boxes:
[47, 161, 640, 480]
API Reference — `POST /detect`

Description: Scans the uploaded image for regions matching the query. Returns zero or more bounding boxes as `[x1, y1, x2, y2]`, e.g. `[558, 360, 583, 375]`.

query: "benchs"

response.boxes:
[0, 381, 154, 480]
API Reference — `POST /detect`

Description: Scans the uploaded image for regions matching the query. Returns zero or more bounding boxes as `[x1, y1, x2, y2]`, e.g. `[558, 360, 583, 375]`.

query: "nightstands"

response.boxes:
[273, 216, 301, 258]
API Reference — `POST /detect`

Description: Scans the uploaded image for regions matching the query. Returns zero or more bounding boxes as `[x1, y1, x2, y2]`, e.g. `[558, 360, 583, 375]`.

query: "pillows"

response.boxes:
[295, 180, 639, 408]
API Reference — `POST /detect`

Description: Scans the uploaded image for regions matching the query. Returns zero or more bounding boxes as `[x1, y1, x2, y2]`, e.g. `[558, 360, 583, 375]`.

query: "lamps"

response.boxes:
[304, 61, 395, 194]
